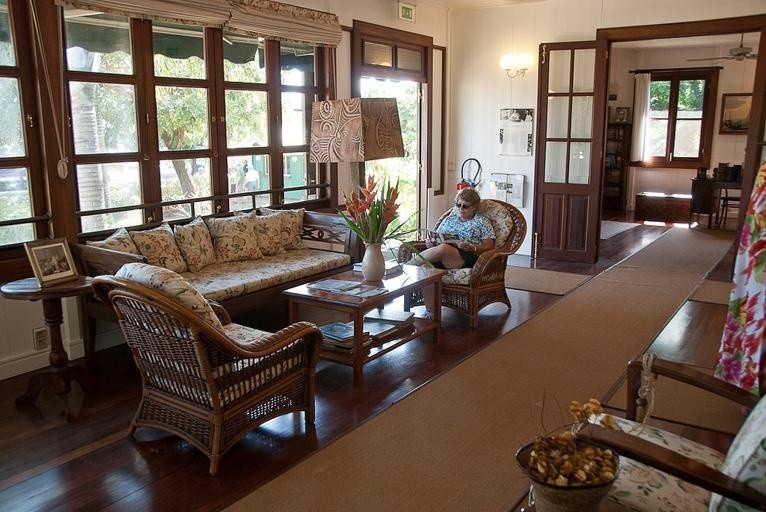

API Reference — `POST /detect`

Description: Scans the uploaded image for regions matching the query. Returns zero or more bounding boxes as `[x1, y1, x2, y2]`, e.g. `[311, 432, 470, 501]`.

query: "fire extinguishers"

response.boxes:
[457, 158, 482, 193]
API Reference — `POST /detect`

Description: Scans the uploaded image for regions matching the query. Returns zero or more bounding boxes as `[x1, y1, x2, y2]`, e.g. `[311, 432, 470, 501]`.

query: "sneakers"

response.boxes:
[421, 311, 431, 319]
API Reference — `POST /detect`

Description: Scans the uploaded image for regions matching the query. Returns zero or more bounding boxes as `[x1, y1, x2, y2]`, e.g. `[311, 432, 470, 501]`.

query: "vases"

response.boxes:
[362, 241, 385, 281]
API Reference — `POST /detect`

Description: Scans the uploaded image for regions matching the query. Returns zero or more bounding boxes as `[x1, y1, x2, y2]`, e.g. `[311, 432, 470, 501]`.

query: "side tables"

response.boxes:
[0, 276, 95, 422]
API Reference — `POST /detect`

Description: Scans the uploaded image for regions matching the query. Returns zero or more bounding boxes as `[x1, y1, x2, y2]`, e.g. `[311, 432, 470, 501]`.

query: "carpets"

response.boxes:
[504, 264, 595, 295]
[688, 278, 731, 306]
[224, 222, 737, 512]
[600, 220, 642, 240]
[603, 360, 751, 434]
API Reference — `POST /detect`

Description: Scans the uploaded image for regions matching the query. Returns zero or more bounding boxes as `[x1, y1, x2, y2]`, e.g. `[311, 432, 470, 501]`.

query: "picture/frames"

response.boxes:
[24, 237, 78, 288]
[615, 107, 630, 122]
[718, 93, 753, 135]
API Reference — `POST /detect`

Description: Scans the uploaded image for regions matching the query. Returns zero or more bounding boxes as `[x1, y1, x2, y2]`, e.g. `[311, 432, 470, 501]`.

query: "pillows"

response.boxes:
[86, 206, 305, 273]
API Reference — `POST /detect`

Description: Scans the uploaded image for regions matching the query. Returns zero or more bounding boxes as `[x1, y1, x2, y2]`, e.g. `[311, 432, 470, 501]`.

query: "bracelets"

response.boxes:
[474, 246, 477, 251]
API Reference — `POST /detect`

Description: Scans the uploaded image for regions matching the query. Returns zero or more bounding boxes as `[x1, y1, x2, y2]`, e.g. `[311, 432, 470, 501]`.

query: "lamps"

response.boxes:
[307, 98, 407, 216]
[500, 52, 531, 78]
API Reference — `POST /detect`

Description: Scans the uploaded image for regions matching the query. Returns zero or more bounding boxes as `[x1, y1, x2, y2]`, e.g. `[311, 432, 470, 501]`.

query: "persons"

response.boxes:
[616, 110, 628, 123]
[405, 189, 497, 322]
[193, 166, 209, 180]
[510, 110, 521, 119]
[524, 110, 533, 122]
[229, 158, 261, 198]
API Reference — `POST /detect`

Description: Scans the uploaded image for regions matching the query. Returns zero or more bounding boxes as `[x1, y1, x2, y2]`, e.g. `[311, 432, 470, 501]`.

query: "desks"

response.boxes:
[688, 178, 743, 230]
[281, 261, 449, 387]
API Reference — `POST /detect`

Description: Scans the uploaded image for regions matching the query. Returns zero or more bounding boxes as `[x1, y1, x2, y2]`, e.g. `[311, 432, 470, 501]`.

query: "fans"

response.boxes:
[686, 33, 758, 64]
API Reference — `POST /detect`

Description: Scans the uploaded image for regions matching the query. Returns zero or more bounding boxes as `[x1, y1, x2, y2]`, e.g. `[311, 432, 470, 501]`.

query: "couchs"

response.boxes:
[71, 211, 363, 365]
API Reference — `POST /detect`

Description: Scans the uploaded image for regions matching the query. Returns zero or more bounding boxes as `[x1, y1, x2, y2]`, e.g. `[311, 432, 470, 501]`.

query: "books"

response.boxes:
[427, 230, 461, 247]
[306, 278, 362, 293]
[340, 285, 378, 296]
[319, 307, 417, 355]
[601, 128, 627, 198]
[355, 287, 389, 298]
[352, 259, 404, 276]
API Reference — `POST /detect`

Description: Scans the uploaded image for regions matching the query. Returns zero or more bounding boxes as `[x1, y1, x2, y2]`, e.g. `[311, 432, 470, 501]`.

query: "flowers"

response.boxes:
[332, 174, 403, 244]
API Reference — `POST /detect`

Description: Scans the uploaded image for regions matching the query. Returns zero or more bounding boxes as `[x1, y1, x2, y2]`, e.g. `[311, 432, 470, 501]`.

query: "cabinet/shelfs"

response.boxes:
[602, 123, 631, 211]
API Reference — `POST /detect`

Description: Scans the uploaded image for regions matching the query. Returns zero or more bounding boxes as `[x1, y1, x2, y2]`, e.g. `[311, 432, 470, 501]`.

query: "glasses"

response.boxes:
[455, 204, 469, 209]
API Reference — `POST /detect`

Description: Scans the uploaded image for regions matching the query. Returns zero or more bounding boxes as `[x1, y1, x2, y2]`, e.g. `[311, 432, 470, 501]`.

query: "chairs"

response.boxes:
[572, 354, 766, 511]
[398, 199, 527, 328]
[92, 262, 324, 473]
[719, 189, 741, 226]
